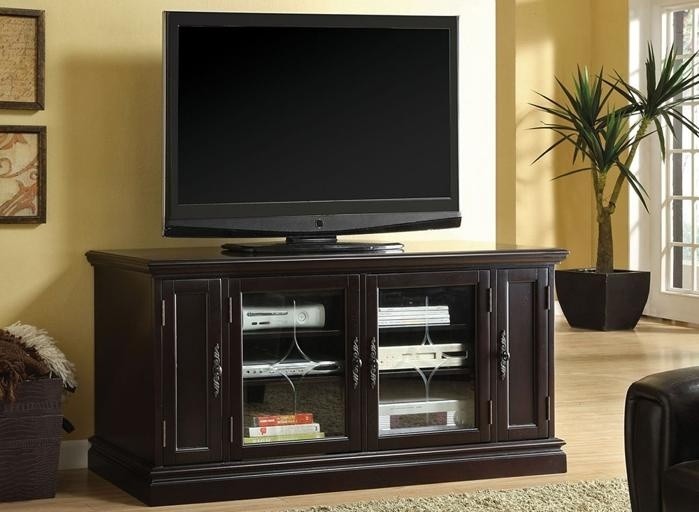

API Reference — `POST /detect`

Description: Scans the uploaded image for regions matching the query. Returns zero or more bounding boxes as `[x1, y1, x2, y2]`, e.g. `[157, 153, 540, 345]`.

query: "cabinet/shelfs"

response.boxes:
[85, 248, 570, 507]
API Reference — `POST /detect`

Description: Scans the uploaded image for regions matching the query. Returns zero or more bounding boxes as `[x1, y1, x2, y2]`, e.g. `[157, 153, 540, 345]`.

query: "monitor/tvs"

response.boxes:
[159, 9, 463, 258]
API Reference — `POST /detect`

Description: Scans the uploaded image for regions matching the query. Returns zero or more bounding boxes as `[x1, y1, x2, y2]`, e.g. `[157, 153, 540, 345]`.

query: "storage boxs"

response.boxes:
[0, 377, 63, 505]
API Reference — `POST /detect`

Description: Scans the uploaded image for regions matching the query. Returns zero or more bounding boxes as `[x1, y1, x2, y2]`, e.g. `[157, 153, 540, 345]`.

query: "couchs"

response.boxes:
[624, 366, 699, 512]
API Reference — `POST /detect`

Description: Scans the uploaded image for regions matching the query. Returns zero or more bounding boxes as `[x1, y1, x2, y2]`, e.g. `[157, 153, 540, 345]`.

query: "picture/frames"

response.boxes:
[0, 7, 44, 110]
[0, 125, 47, 225]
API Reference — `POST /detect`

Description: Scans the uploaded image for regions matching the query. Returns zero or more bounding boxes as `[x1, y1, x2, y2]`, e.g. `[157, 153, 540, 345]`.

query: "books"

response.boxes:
[243, 407, 326, 444]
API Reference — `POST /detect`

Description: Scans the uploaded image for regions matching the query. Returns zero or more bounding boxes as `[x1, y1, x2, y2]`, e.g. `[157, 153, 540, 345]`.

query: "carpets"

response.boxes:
[280, 476, 632, 512]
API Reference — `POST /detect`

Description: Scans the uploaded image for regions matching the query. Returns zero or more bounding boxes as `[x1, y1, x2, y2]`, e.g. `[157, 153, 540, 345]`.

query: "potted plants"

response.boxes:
[524, 41, 699, 331]
